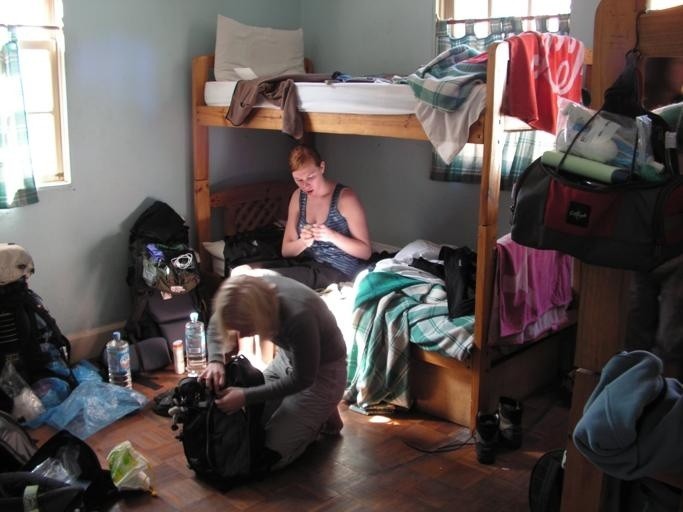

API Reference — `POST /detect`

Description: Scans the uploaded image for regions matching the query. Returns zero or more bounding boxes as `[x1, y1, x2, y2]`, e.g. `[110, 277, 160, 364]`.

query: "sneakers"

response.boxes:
[497, 396, 524, 450]
[473, 410, 499, 464]
[322, 407, 343, 434]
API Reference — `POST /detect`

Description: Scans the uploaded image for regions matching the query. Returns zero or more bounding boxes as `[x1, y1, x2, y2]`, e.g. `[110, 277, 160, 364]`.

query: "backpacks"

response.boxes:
[175, 356, 264, 491]
[120, 294, 211, 359]
[0, 275, 77, 412]
[529, 448, 683, 512]
[127, 201, 201, 294]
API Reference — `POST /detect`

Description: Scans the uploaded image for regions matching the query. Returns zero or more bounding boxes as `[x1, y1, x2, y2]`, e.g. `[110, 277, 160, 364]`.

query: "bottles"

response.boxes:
[185, 311, 206, 378]
[106, 331, 133, 391]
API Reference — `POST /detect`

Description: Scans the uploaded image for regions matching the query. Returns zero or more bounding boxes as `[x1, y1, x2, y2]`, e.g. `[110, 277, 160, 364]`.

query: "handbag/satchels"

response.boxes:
[439, 247, 477, 318]
[224, 224, 310, 280]
[511, 154, 681, 272]
[102, 337, 171, 381]
[0, 411, 149, 512]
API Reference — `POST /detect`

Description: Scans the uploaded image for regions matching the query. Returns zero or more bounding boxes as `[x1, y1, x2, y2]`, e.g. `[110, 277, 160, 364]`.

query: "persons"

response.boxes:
[195, 266, 349, 472]
[263, 145, 373, 293]
